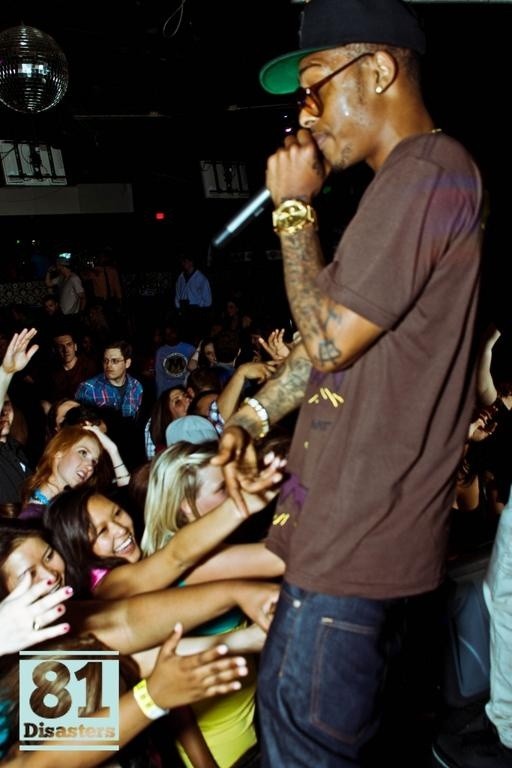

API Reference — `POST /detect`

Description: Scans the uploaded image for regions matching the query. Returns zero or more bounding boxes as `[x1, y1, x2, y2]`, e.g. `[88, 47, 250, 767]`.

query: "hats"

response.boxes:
[165, 415, 219, 447]
[259, 0, 427, 96]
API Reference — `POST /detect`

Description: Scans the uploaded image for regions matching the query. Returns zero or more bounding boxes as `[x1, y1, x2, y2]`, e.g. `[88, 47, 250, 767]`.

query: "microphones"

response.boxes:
[212, 187, 272, 252]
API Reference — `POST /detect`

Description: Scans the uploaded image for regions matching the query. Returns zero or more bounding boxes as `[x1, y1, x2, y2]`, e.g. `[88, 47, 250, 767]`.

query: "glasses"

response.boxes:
[293, 52, 376, 117]
[103, 358, 124, 364]
